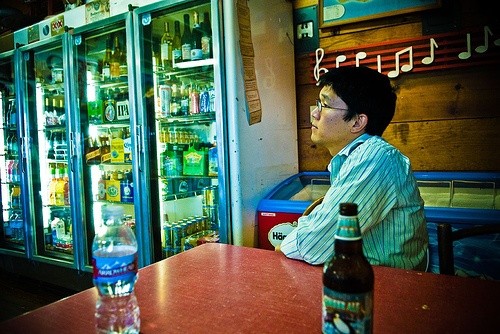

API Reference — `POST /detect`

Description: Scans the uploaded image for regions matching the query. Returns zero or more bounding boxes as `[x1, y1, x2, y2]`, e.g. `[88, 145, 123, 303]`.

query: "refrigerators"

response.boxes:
[257, 169, 500, 280]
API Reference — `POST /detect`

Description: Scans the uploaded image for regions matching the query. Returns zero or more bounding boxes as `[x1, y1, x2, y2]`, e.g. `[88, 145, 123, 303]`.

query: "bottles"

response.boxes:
[320, 203, 375, 334]
[0, 9, 221, 247]
[91, 203, 142, 334]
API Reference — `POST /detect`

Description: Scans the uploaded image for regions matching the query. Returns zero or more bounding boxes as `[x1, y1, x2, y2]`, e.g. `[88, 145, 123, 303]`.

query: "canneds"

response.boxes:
[189, 91, 210, 114]
[164, 185, 217, 258]
[159, 85, 173, 117]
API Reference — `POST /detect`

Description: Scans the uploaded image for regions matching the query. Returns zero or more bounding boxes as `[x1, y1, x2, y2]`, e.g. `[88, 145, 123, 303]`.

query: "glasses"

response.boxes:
[315, 98, 348, 112]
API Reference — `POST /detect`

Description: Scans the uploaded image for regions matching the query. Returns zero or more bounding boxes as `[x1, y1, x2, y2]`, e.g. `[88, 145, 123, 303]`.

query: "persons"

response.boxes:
[280, 65, 430, 273]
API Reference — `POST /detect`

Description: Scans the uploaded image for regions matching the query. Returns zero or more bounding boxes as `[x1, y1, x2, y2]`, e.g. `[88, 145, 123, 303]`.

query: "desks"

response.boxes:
[0, 243, 500, 334]
